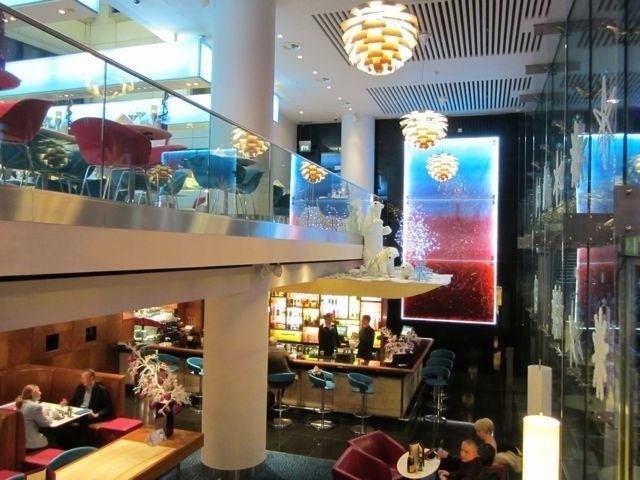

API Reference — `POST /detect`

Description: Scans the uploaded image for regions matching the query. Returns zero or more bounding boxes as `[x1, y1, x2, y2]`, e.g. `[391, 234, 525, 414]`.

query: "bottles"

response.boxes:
[406, 439, 427, 475]
[271, 292, 380, 344]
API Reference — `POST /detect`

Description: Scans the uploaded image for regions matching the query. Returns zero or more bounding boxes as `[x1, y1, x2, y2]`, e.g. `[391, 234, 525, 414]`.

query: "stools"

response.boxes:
[187, 357, 204, 415]
[421, 349, 457, 423]
[152, 354, 180, 373]
[266, 351, 296, 429]
[347, 372, 376, 435]
[308, 369, 335, 430]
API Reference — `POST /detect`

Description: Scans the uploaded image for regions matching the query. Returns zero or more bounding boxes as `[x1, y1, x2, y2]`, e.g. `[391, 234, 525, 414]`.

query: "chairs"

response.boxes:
[57, 117, 266, 219]
[273, 181, 290, 225]
[0, 359, 203, 480]
[0, 69, 57, 187]
[330, 427, 522, 480]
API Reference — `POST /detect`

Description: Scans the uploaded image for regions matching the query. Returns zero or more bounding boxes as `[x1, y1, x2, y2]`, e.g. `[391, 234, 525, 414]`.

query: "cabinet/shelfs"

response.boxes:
[269, 292, 382, 345]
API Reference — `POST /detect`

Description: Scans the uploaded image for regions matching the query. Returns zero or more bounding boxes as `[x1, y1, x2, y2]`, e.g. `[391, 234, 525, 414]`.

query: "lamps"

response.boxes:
[526, 358, 552, 416]
[339, 0, 420, 77]
[400, 31, 449, 152]
[300, 161, 327, 185]
[426, 152, 460, 182]
[521, 413, 562, 480]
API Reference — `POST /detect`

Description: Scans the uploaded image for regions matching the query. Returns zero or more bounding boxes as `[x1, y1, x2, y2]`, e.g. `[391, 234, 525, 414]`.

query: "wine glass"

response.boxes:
[127, 103, 160, 126]
[331, 187, 342, 198]
[54, 109, 62, 132]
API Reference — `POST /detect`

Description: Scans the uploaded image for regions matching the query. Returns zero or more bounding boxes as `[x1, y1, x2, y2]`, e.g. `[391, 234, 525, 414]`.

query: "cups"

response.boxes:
[135, 307, 172, 343]
[50, 406, 74, 421]
[282, 345, 382, 364]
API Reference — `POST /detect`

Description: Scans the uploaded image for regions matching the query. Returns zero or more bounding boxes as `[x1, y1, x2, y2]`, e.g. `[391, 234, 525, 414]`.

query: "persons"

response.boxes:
[14, 384, 59, 454]
[437, 438, 484, 480]
[433, 416, 498, 473]
[268, 336, 291, 403]
[56, 367, 113, 450]
[354, 314, 375, 360]
[319, 312, 343, 358]
